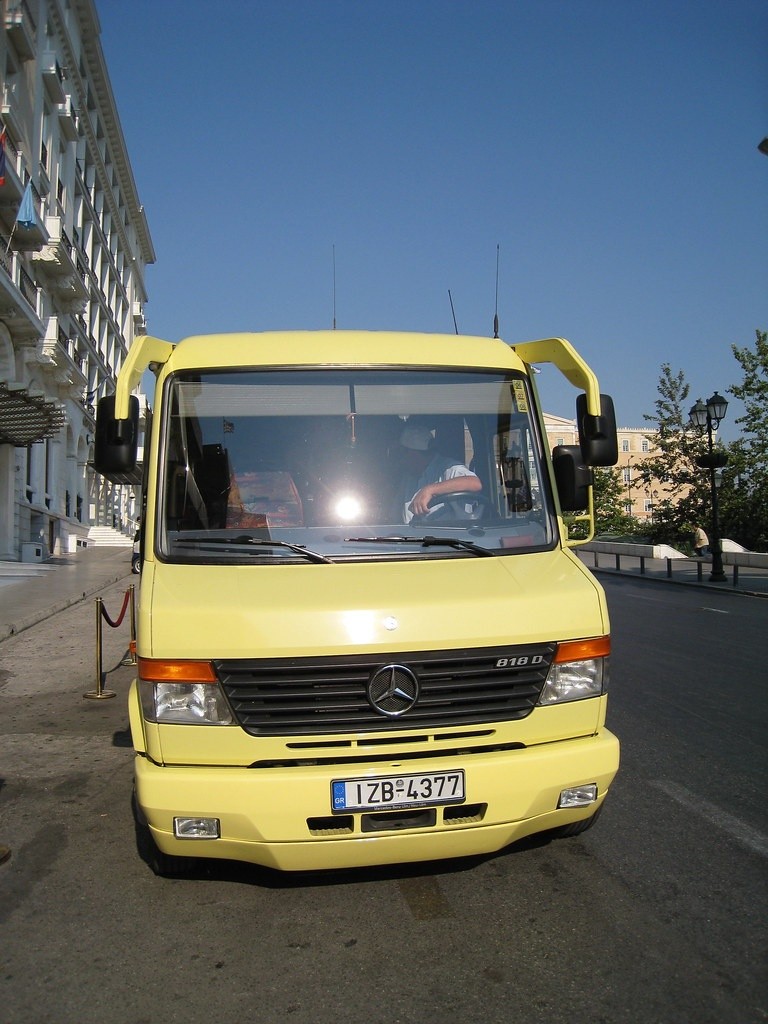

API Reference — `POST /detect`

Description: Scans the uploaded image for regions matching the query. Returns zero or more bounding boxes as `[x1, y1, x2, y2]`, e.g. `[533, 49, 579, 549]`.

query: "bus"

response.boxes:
[95, 330, 620, 874]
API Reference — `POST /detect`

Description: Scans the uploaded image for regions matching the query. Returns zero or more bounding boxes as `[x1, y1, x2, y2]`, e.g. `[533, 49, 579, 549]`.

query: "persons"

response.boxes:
[691, 522, 709, 556]
[131, 515, 142, 566]
[382, 424, 482, 525]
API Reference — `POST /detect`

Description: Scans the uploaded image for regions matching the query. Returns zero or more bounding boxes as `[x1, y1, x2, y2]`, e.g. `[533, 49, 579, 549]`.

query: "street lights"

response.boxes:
[687, 391, 730, 581]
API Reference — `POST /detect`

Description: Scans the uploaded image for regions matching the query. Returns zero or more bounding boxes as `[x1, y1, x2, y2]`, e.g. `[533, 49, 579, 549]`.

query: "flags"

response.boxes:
[0, 132, 6, 186]
[16, 181, 37, 230]
